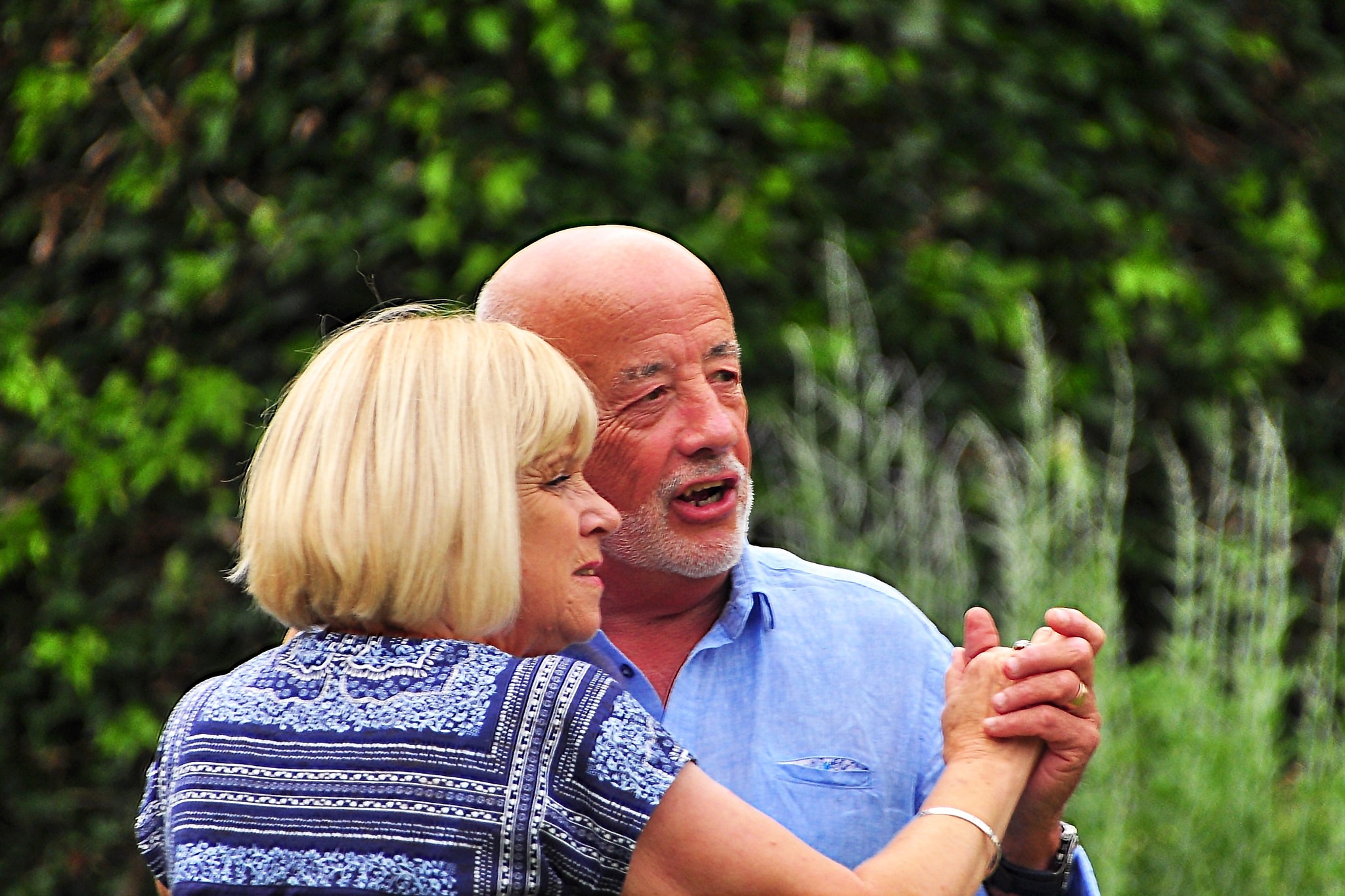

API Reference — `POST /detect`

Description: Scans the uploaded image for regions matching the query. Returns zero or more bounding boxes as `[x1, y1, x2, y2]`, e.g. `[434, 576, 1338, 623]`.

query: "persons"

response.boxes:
[140, 226, 1107, 896]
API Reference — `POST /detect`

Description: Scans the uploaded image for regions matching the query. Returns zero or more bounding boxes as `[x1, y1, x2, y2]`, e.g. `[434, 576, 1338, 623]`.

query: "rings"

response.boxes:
[1010, 639, 1031, 652]
[1064, 680, 1088, 711]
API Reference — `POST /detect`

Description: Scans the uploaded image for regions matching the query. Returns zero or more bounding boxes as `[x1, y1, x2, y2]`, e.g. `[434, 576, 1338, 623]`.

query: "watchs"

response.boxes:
[997, 816, 1079, 881]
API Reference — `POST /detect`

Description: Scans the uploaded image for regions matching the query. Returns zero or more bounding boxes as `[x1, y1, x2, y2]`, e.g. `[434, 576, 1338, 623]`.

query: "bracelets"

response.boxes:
[913, 804, 1003, 881]
